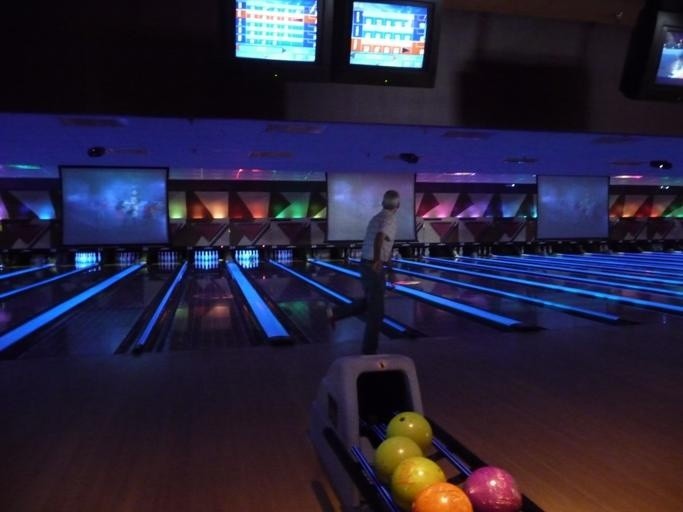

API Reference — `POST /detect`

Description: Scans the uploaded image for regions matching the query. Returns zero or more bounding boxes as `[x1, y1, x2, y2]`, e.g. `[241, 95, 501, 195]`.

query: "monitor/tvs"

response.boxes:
[333, 0, 438, 88]
[219, 0, 326, 80]
[617, 0, 683, 105]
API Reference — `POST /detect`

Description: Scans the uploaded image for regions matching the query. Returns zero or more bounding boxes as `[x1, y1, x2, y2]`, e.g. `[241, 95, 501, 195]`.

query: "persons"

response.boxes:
[115, 186, 166, 227]
[326, 190, 400, 355]
